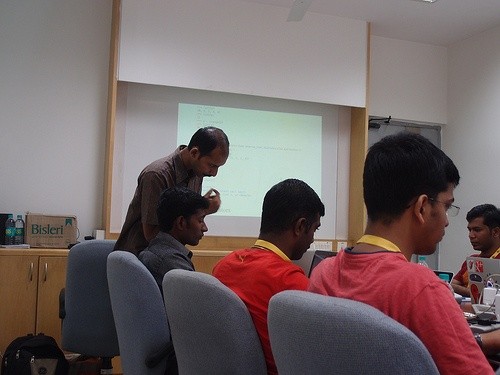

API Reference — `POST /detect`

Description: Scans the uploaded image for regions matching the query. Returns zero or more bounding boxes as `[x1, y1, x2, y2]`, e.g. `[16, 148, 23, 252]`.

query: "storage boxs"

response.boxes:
[24, 214, 77, 248]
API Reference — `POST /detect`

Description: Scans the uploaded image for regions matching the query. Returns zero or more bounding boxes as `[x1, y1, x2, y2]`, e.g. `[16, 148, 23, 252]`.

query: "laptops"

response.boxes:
[308, 250, 337, 279]
[466, 257, 500, 303]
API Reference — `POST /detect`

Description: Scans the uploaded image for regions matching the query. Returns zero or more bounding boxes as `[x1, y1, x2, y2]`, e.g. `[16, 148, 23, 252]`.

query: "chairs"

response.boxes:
[59, 239, 454, 375]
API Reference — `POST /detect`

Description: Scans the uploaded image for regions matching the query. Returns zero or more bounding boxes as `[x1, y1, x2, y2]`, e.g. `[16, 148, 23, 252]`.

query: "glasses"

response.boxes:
[429, 198, 460, 217]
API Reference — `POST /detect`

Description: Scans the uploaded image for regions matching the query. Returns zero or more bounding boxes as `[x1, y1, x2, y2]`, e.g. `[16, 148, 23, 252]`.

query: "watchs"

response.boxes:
[474, 333, 482, 347]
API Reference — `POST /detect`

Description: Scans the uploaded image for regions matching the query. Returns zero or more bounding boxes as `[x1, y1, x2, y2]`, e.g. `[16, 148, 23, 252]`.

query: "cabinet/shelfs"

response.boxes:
[0, 246, 229, 353]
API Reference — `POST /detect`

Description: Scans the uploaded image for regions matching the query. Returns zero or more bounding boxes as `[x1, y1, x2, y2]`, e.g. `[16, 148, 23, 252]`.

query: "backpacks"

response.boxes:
[0, 332, 70, 375]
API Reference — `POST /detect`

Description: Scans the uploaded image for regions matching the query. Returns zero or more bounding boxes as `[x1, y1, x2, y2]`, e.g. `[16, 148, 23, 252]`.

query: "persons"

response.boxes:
[310, 133, 496, 375]
[137, 188, 208, 302]
[113, 127, 230, 263]
[451, 204, 500, 297]
[213, 179, 325, 375]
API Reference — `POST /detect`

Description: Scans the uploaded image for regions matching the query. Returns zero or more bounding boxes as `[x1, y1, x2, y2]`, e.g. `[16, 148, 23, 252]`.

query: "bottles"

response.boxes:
[439, 274, 454, 296]
[15, 215, 25, 245]
[417, 256, 429, 267]
[5, 214, 15, 245]
[460, 297, 472, 313]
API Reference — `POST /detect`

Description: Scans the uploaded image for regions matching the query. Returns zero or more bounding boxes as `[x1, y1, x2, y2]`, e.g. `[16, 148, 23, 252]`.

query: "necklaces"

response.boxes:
[255, 240, 292, 262]
[356, 235, 401, 252]
